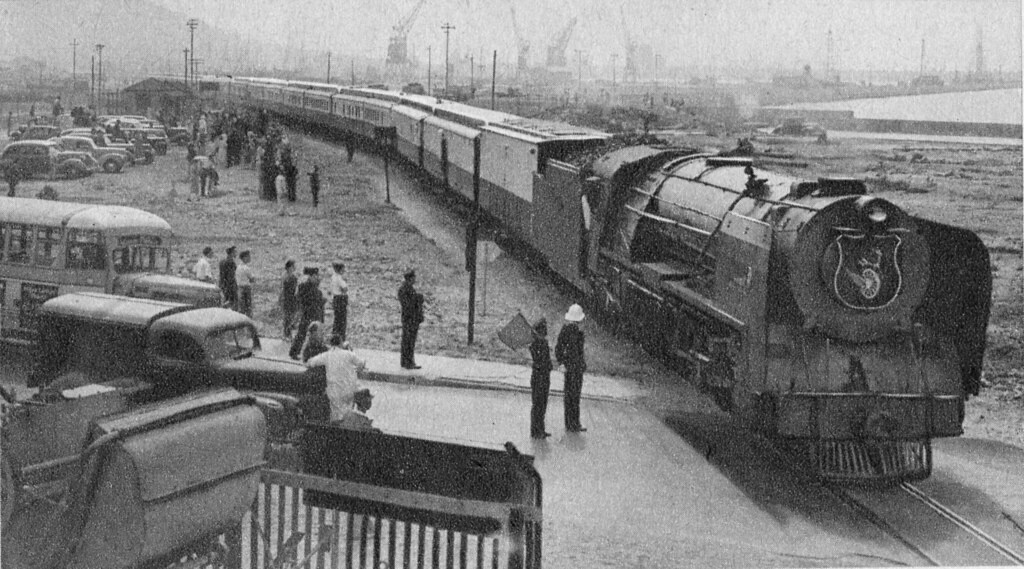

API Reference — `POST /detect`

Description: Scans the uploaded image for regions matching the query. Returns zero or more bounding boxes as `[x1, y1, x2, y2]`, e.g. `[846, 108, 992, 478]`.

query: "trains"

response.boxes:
[179, 75, 992, 488]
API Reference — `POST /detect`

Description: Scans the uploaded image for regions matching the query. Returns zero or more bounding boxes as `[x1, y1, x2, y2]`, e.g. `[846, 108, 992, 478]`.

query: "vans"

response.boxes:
[0, 196, 225, 358]
[28, 290, 372, 426]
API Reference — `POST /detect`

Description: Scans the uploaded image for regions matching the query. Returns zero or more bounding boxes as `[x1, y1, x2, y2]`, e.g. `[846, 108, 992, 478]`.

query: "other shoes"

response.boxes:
[567, 424, 587, 432]
[401, 362, 422, 369]
[531, 430, 551, 438]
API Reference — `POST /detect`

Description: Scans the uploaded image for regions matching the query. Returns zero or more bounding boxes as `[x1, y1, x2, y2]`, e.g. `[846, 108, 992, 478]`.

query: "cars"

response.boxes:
[0, 115, 188, 180]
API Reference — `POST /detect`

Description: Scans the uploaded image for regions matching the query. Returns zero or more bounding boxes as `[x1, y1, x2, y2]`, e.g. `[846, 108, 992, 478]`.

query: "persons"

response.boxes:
[282, 260, 296, 342]
[290, 268, 326, 359]
[236, 250, 254, 317]
[189, 158, 200, 203]
[398, 271, 424, 369]
[555, 304, 587, 432]
[308, 165, 318, 207]
[307, 336, 363, 421]
[192, 247, 215, 284]
[29, 105, 37, 122]
[219, 245, 237, 308]
[282, 161, 298, 202]
[340, 389, 381, 539]
[331, 262, 349, 341]
[53, 94, 64, 115]
[303, 322, 326, 363]
[530, 318, 553, 438]
[344, 130, 356, 162]
[275, 171, 288, 214]
[72, 104, 292, 196]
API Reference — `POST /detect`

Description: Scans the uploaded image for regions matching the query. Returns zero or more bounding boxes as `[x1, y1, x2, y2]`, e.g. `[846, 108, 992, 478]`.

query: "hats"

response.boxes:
[285, 259, 295, 268]
[565, 303, 585, 321]
[531, 318, 547, 333]
[226, 246, 236, 255]
[354, 388, 375, 401]
[403, 268, 416, 276]
